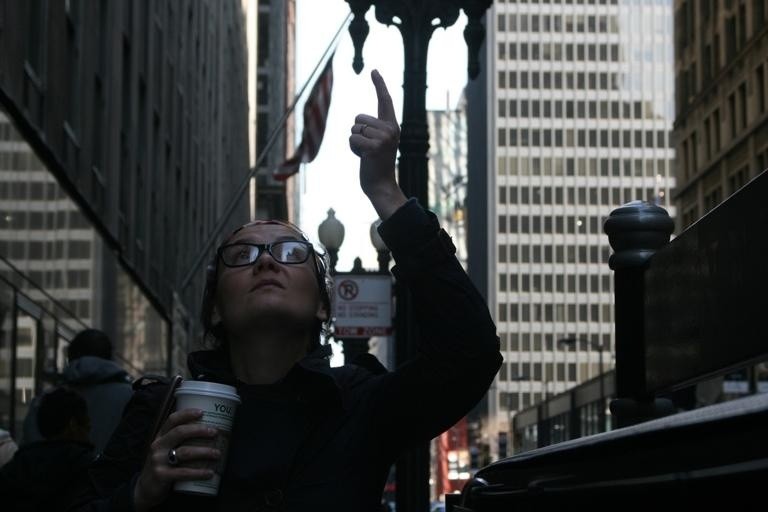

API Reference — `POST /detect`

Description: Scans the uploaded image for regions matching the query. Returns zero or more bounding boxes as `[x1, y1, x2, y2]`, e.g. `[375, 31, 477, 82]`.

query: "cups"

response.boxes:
[174, 380, 241, 495]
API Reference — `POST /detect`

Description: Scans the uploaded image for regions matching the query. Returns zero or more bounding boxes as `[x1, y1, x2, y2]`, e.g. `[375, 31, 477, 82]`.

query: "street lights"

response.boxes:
[557, 337, 608, 432]
[317, 205, 397, 366]
[512, 374, 553, 447]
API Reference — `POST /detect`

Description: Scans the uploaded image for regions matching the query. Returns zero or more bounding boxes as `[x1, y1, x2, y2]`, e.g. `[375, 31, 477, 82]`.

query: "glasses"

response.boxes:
[217, 241, 318, 271]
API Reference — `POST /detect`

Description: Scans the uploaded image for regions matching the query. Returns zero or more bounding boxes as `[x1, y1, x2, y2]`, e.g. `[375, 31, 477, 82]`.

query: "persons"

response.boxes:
[84, 65, 508, 510]
[22, 323, 136, 512]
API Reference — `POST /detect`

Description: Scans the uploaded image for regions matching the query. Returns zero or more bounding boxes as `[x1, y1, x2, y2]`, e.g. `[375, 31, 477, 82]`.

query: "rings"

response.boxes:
[166, 449, 178, 466]
[359, 123, 367, 136]
